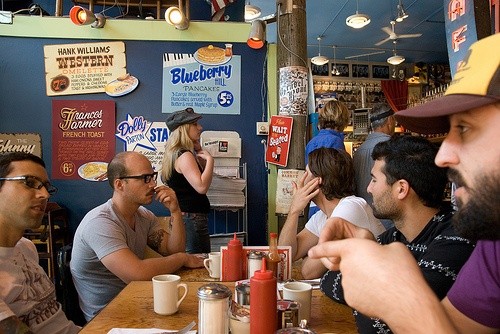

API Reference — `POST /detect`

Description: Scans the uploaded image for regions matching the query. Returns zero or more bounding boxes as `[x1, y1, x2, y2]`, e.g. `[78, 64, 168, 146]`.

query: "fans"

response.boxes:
[374, 20, 422, 48]
[345, 42, 427, 62]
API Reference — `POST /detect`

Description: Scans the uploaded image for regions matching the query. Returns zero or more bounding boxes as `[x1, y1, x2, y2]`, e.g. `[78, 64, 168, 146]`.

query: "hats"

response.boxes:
[394, 32, 499, 136]
[165, 108, 203, 132]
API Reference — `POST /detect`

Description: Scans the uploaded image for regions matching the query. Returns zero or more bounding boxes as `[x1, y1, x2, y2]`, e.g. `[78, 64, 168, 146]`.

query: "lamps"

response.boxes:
[245, 0, 262, 19]
[0, 7, 43, 24]
[311, 37, 330, 65]
[386, 53, 406, 66]
[345, 0, 371, 29]
[331, 46, 340, 75]
[394, 0, 408, 22]
[164, 7, 190, 30]
[69, 6, 106, 28]
[246, 12, 277, 49]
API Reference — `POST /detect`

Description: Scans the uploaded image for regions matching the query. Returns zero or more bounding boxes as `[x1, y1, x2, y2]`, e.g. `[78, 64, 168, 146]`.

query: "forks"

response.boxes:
[153, 320, 196, 334]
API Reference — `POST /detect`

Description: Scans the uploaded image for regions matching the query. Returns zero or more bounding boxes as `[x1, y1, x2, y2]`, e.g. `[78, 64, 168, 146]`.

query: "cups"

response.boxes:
[151, 274, 189, 316]
[236, 282, 250, 305]
[276, 298, 302, 327]
[203, 252, 221, 279]
[276, 327, 316, 334]
[283, 282, 313, 324]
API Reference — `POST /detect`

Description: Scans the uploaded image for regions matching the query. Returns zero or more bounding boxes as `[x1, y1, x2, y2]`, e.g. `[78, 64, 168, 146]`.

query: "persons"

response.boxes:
[304, 100, 349, 219]
[278, 148, 387, 280]
[350, 103, 395, 231]
[0, 151, 82, 334]
[69, 151, 209, 323]
[161, 108, 214, 254]
[320, 132, 477, 334]
[309, 33, 500, 334]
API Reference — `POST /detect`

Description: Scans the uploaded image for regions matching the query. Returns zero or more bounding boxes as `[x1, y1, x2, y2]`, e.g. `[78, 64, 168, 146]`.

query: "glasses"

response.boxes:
[118, 172, 158, 184]
[0, 175, 58, 196]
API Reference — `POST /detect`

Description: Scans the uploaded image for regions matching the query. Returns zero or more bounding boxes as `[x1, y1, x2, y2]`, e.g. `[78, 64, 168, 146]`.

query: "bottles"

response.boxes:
[246, 249, 266, 278]
[344, 132, 366, 156]
[267, 232, 282, 283]
[406, 81, 452, 110]
[197, 282, 231, 334]
[313, 80, 385, 113]
[443, 182, 451, 199]
[250, 256, 277, 334]
[227, 232, 242, 281]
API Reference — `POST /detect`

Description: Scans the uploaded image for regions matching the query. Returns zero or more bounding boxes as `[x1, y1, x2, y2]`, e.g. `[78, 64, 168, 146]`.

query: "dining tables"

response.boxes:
[76, 266, 357, 334]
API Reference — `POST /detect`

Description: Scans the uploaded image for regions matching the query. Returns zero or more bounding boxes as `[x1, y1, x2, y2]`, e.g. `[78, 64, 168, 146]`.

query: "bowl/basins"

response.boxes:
[228, 304, 250, 334]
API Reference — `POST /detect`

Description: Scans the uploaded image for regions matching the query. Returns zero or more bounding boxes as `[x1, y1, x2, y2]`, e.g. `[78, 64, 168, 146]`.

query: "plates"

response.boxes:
[78, 162, 109, 182]
[193, 48, 232, 66]
[105, 76, 138, 97]
